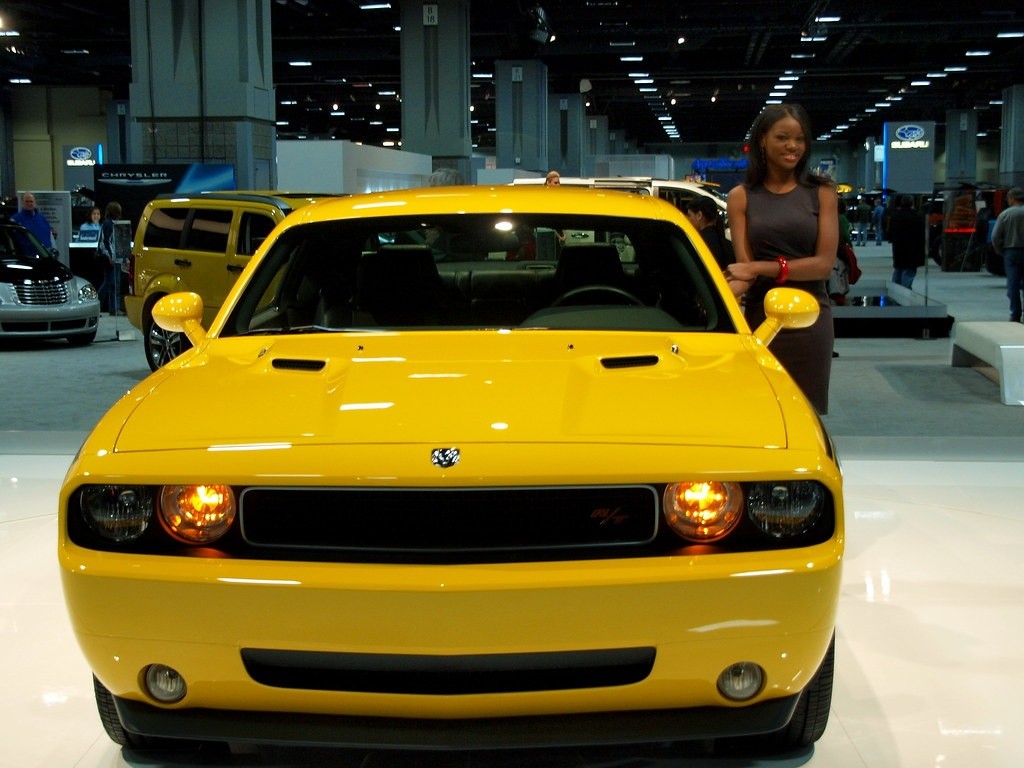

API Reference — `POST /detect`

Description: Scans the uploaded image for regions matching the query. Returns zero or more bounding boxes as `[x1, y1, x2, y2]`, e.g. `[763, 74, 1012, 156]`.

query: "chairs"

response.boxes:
[358, 245, 463, 326]
[542, 247, 646, 308]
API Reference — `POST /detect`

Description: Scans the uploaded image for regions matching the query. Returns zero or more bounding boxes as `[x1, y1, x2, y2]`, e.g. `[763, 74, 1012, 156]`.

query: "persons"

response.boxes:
[835, 199, 862, 283]
[890, 193, 919, 289]
[991, 188, 1024, 322]
[687, 197, 735, 272]
[10, 192, 59, 265]
[543, 171, 567, 244]
[723, 103, 840, 414]
[80, 201, 126, 316]
[427, 167, 465, 187]
[853, 197, 884, 246]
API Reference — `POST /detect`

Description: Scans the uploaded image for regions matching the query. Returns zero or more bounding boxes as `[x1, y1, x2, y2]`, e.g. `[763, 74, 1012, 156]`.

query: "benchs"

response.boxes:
[433, 262, 651, 326]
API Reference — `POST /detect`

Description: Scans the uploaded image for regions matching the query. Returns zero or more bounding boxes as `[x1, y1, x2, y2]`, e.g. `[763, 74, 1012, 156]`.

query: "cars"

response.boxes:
[366, 177, 736, 303]
[57, 181, 850, 766]
[0, 214, 102, 350]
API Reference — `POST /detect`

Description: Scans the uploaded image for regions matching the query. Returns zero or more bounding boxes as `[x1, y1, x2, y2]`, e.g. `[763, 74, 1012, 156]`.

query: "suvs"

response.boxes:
[124, 189, 365, 376]
